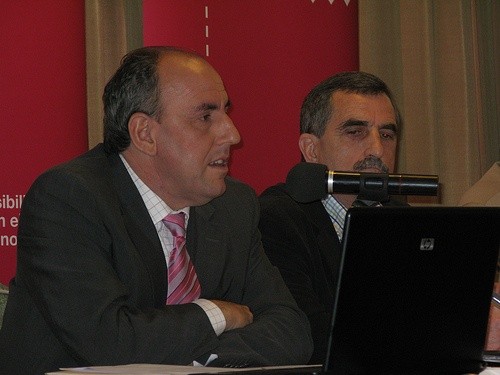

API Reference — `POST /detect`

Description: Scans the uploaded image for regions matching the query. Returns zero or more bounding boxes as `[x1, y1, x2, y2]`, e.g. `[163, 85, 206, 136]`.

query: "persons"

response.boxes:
[0, 46, 314, 374]
[259, 72, 500, 352]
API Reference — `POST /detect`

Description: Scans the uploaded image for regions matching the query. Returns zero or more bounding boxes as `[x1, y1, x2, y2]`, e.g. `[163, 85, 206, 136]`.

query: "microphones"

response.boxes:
[286, 161, 440, 204]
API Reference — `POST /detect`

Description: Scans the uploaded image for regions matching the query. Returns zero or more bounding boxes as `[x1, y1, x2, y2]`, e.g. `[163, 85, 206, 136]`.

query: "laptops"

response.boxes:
[188, 205, 499, 375]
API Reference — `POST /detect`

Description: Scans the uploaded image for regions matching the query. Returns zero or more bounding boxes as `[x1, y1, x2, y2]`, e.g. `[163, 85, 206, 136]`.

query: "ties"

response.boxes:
[162, 213, 201, 303]
